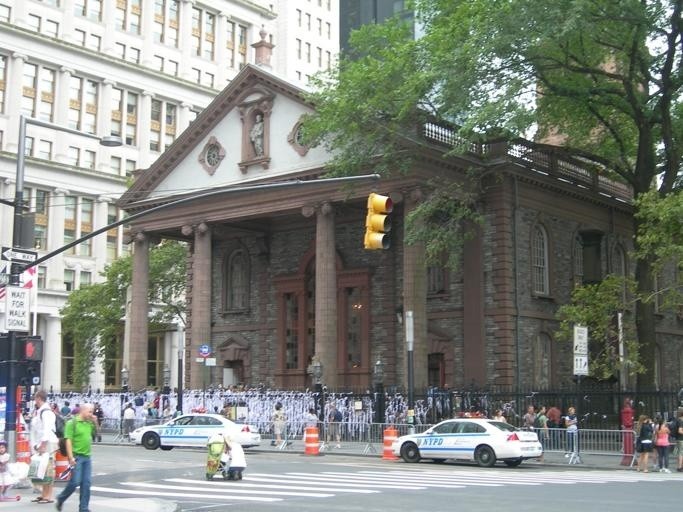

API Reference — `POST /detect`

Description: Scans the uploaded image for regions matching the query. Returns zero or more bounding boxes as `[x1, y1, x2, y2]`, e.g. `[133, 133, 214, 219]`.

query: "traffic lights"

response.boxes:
[14, 336, 43, 362]
[362, 192, 393, 250]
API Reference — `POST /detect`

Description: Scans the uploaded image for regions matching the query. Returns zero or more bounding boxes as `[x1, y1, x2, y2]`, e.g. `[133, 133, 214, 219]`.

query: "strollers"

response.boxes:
[204, 433, 230, 480]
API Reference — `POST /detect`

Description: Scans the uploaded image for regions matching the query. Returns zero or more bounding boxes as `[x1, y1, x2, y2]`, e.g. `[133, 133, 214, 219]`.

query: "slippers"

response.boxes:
[32, 497, 53, 503]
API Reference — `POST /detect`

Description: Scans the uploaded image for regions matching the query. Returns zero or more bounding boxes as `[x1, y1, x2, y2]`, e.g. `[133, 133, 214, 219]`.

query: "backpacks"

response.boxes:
[335, 409, 341, 421]
[40, 408, 65, 438]
[59, 418, 76, 455]
[534, 414, 544, 428]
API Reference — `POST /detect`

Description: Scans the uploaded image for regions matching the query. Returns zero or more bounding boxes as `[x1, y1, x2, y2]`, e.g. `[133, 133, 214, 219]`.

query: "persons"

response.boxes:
[222, 434, 247, 479]
[636, 406, 683, 472]
[0, 439, 11, 500]
[54, 403, 101, 512]
[24, 402, 102, 455]
[471, 401, 485, 416]
[324, 403, 342, 448]
[494, 409, 507, 423]
[121, 393, 182, 438]
[209, 384, 249, 413]
[524, 406, 579, 462]
[30, 390, 58, 503]
[306, 409, 319, 427]
[271, 405, 284, 446]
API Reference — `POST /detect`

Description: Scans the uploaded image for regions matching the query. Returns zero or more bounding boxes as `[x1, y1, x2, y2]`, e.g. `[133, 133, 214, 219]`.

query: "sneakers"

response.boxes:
[56, 493, 62, 510]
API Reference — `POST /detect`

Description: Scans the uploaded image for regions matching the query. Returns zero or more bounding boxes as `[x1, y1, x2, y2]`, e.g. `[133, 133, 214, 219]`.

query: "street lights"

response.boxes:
[312, 357, 324, 421]
[120, 364, 129, 395]
[371, 355, 385, 423]
[404, 309, 414, 435]
[4, 115, 123, 461]
[176, 322, 184, 412]
[161, 364, 170, 395]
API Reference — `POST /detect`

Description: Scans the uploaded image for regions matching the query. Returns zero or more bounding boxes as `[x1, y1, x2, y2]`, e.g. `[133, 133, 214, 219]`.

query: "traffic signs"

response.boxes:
[573, 355, 588, 376]
[0, 246, 37, 267]
[572, 324, 588, 354]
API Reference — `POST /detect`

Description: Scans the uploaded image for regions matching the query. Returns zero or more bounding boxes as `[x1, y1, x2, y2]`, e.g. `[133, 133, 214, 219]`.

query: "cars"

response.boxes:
[389, 412, 543, 467]
[129, 407, 261, 451]
